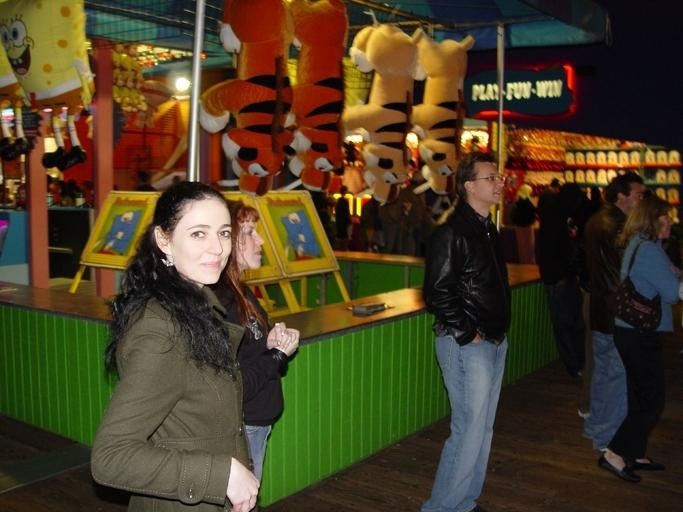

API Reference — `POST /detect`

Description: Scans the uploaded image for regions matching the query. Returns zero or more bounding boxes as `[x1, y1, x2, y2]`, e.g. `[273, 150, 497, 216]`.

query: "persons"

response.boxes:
[419, 149, 514, 507]
[311, 167, 441, 259]
[209, 198, 302, 491]
[87, 177, 263, 511]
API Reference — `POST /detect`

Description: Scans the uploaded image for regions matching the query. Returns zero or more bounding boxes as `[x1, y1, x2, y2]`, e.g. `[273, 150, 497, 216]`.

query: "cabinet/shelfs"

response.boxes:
[563, 146, 681, 188]
[46, 204, 94, 256]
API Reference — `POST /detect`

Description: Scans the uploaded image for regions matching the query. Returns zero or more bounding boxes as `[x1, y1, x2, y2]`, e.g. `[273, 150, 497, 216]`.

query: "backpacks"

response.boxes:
[579, 240, 621, 298]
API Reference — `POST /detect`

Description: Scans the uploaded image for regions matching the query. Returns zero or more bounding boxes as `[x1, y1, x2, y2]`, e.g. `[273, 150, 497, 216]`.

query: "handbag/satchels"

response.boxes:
[609, 241, 661, 331]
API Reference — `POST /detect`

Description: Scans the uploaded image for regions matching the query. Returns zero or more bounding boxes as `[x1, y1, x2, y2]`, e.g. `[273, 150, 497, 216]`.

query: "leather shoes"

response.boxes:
[631, 456, 665, 471]
[597, 451, 642, 484]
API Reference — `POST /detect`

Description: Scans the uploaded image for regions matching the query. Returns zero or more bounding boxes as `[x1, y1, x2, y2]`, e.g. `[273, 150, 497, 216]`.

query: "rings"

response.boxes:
[274, 322, 281, 326]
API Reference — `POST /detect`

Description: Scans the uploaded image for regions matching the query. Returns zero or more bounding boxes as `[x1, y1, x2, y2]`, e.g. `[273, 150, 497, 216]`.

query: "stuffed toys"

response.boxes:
[0, 1, 477, 215]
